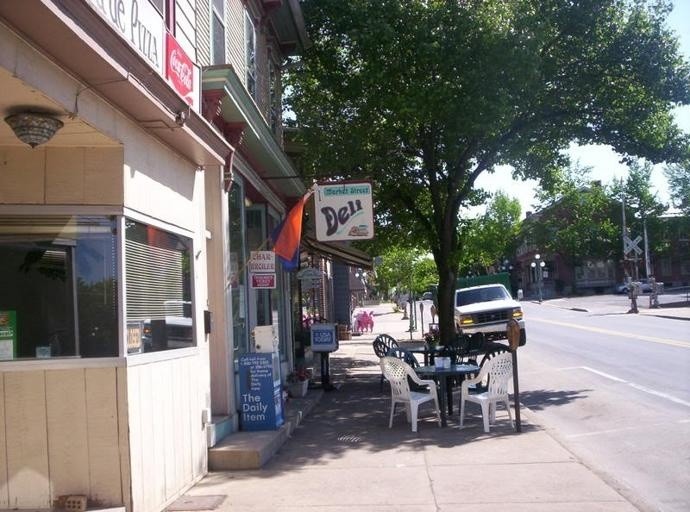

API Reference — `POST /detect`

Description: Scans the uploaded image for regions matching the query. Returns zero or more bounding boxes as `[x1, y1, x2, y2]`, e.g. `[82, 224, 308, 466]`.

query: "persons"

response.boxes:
[353, 309, 364, 332]
[363, 311, 369, 331]
[368, 310, 375, 333]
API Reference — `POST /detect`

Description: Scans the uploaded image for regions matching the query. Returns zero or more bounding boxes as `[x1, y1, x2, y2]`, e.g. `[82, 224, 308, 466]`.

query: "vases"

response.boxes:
[290, 381, 308, 397]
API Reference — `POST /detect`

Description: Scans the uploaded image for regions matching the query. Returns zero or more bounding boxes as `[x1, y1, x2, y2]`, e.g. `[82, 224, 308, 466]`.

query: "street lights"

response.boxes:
[531, 253, 546, 302]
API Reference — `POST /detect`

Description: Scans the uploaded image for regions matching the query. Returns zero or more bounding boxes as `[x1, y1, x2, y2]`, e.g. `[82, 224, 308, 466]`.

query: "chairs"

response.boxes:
[374, 332, 515, 432]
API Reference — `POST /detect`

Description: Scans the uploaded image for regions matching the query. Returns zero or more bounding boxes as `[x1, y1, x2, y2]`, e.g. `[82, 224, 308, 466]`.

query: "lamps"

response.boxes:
[4, 114, 64, 148]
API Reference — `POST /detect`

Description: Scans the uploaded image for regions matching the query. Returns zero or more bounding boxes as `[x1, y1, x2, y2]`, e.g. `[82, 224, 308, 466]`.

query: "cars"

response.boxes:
[454, 283, 525, 346]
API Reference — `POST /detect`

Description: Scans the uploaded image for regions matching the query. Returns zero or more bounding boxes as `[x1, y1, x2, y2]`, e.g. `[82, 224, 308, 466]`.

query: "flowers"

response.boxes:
[286, 365, 310, 384]
[424, 327, 442, 350]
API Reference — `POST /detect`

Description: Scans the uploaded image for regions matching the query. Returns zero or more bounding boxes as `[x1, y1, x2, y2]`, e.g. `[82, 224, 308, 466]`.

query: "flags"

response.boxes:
[269, 193, 307, 273]
